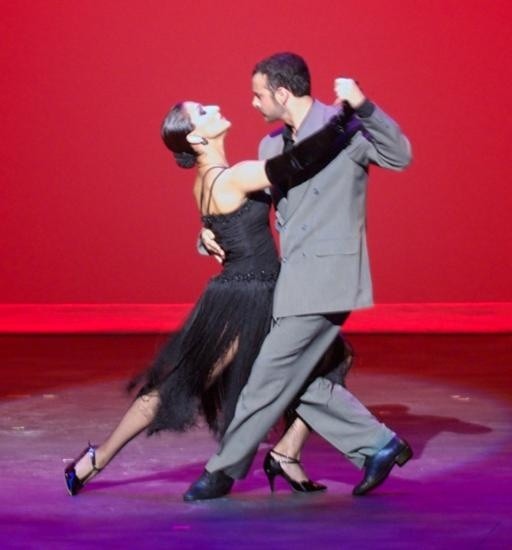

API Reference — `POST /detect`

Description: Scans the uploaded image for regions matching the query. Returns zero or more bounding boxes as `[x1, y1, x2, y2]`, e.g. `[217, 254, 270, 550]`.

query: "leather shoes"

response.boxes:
[352, 435, 412, 496]
[184, 466, 235, 500]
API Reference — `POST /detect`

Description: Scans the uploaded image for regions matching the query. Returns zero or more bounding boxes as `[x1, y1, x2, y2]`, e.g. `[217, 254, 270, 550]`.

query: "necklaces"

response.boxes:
[196, 162, 224, 169]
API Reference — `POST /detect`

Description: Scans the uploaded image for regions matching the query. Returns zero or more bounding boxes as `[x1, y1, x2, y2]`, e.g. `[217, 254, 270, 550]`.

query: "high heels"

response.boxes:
[64, 443, 104, 495]
[263, 450, 326, 493]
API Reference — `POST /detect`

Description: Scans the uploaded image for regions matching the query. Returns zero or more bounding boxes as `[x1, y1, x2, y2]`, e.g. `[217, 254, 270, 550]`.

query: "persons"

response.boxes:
[182, 51, 413, 502]
[64, 82, 363, 497]
